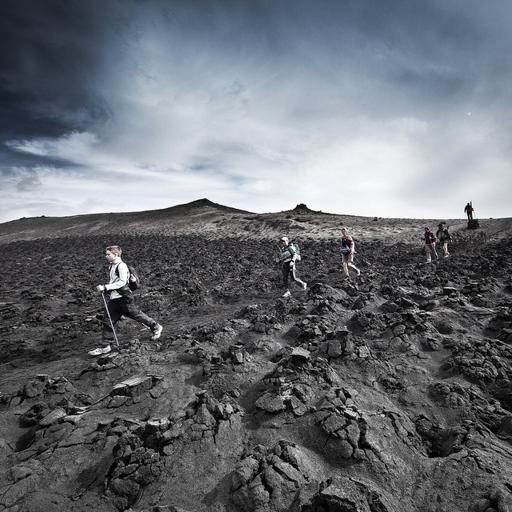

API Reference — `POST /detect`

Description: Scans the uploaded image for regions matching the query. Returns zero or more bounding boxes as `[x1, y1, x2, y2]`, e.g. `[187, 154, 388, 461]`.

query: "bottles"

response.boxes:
[130, 274, 136, 282]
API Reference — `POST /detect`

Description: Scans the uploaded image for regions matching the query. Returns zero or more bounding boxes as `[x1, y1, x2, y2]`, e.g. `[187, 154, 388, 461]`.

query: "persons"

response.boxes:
[436, 224, 452, 258]
[88, 245, 163, 356]
[280, 237, 307, 298]
[464, 203, 472, 221]
[340, 227, 360, 281]
[424, 227, 438, 263]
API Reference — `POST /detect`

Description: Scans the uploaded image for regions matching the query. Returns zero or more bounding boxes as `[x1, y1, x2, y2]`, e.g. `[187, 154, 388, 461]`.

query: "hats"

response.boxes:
[281, 237, 288, 240]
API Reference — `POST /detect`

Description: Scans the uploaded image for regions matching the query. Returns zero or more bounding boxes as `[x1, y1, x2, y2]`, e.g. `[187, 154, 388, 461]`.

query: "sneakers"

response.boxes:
[356, 269, 360, 276]
[344, 276, 349, 281]
[88, 345, 111, 356]
[301, 283, 307, 291]
[150, 323, 163, 340]
[282, 291, 292, 297]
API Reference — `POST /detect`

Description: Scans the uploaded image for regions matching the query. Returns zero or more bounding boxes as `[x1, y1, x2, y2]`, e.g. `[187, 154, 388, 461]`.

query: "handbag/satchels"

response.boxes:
[341, 249, 351, 255]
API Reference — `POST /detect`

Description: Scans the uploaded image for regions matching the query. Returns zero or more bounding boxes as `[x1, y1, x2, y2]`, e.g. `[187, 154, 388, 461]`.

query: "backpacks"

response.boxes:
[287, 243, 301, 262]
[109, 261, 139, 291]
